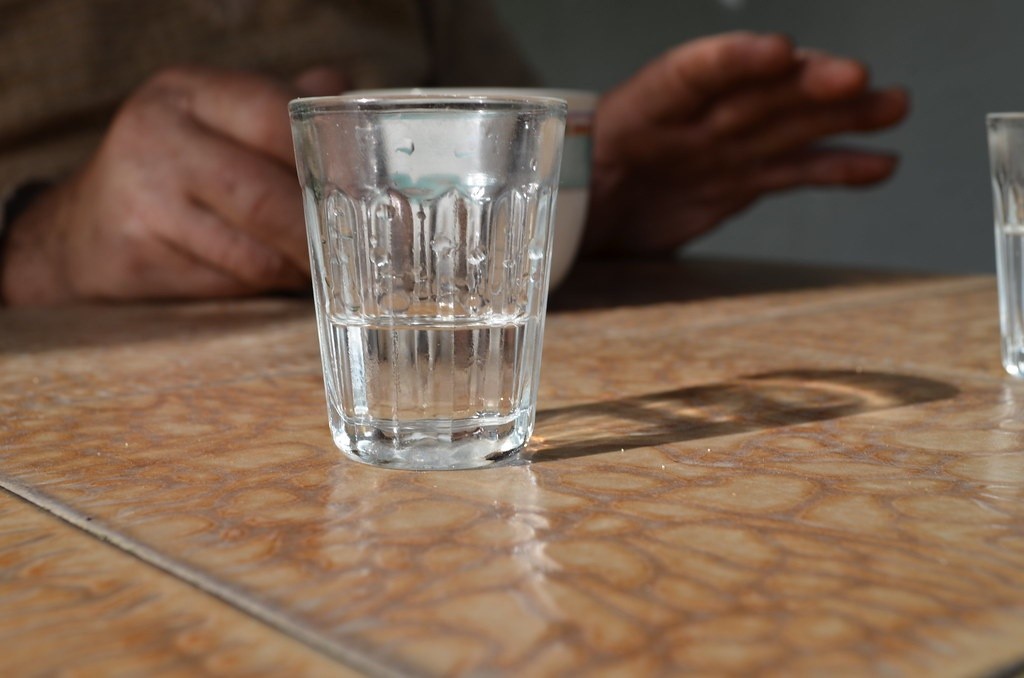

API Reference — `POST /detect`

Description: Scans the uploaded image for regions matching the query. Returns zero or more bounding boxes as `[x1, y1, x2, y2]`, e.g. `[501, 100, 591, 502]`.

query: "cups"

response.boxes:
[287, 95, 569, 471]
[351, 89, 596, 292]
[985, 112, 1024, 382]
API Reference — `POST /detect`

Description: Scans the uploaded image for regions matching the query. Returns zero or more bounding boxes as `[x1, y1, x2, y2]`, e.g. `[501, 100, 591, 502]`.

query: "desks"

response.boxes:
[0, 250, 1024, 678]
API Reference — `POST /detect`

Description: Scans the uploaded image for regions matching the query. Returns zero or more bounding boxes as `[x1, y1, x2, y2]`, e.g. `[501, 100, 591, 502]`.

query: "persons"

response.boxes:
[0, 0, 911, 302]
